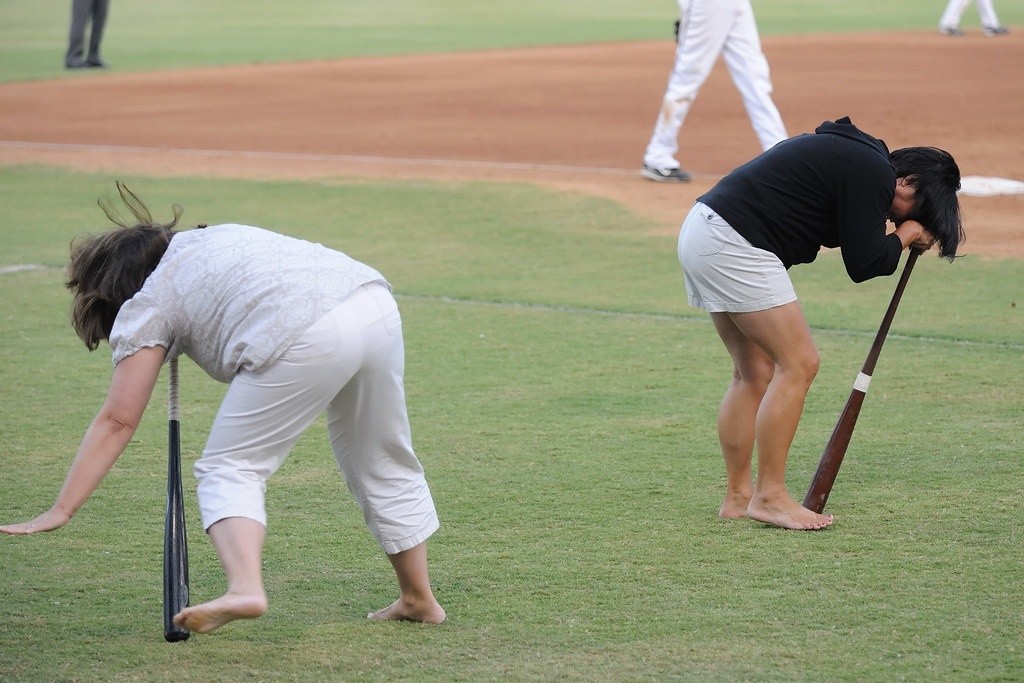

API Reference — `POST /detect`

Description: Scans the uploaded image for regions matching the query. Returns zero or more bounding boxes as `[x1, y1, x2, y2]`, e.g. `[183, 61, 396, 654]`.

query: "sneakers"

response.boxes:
[642, 163, 693, 183]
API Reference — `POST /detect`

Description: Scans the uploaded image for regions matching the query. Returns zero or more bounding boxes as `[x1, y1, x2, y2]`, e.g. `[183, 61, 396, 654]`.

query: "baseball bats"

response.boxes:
[161, 352, 192, 644]
[801, 241, 921, 515]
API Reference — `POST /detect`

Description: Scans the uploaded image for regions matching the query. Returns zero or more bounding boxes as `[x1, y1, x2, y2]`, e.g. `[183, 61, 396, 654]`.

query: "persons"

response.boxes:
[640, 0, 790, 181]
[0, 182, 446, 633]
[63, 0, 109, 69]
[935, 0, 1008, 36]
[678, 117, 965, 528]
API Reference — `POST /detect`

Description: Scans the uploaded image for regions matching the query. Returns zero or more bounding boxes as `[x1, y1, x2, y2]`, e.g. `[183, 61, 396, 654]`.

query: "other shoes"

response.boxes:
[939, 27, 965, 36]
[983, 27, 1010, 35]
